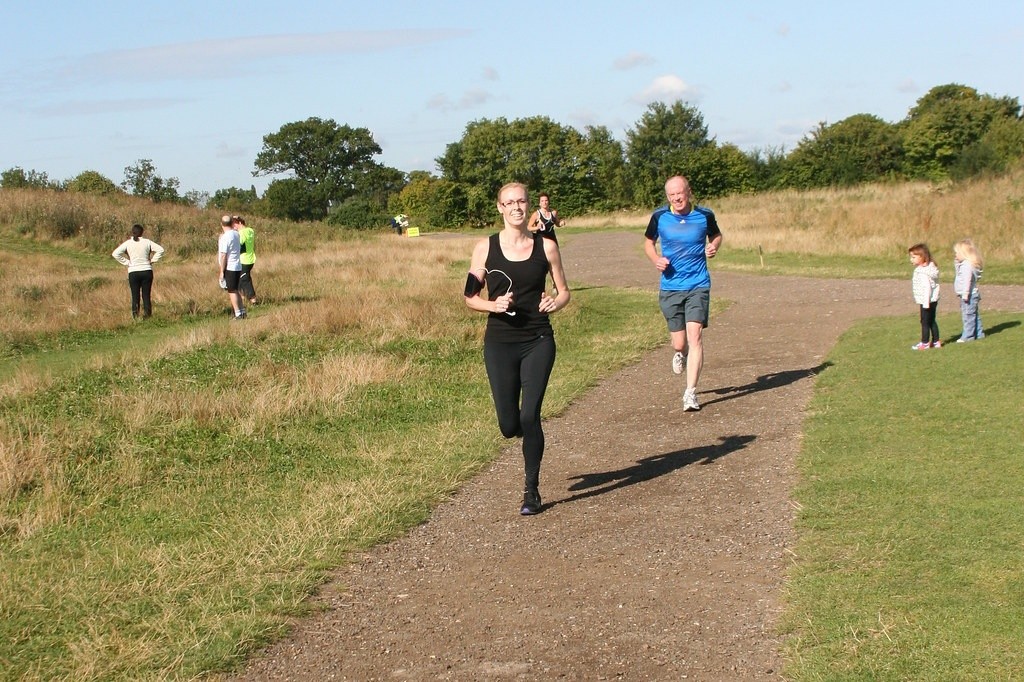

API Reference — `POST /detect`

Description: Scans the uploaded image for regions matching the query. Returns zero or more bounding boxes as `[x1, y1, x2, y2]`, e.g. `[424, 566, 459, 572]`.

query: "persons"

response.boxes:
[464, 182, 572, 517]
[951, 238, 986, 344]
[643, 175, 724, 414]
[390, 213, 408, 235]
[112, 224, 165, 320]
[216, 215, 248, 322]
[526, 192, 567, 294]
[228, 214, 258, 304]
[907, 242, 943, 351]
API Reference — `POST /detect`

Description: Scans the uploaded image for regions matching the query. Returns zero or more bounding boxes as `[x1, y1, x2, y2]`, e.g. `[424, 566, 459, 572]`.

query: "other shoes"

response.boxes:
[957, 339, 966, 343]
[230, 314, 243, 321]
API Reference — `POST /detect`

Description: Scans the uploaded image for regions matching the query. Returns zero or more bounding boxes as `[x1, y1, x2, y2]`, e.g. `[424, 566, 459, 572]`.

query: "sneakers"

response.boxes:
[929, 341, 943, 348]
[911, 342, 930, 350]
[683, 387, 701, 412]
[520, 487, 542, 515]
[672, 345, 689, 374]
